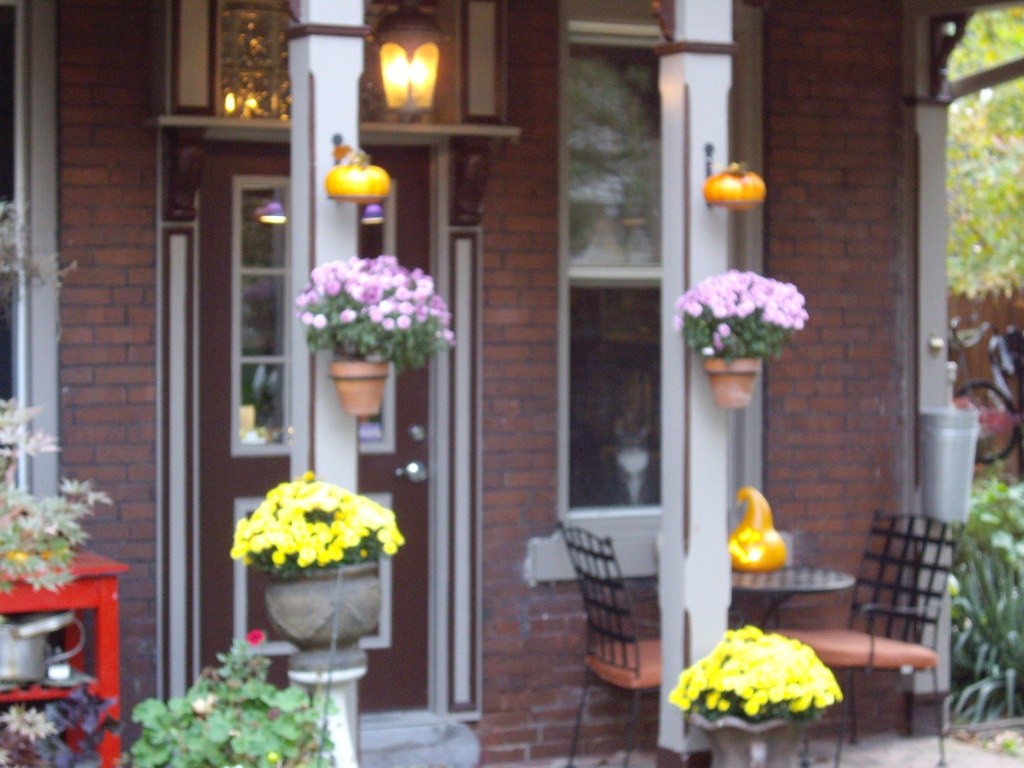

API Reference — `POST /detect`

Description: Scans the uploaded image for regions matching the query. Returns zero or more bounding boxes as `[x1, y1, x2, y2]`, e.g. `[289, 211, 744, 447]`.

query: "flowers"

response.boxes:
[129, 627, 337, 768]
[673, 268, 809, 361]
[669, 624, 846, 723]
[230, 469, 408, 575]
[294, 254, 457, 372]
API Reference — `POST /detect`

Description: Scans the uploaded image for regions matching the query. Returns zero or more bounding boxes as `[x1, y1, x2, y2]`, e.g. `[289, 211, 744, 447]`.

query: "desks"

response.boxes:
[731, 569, 856, 631]
[0, 547, 130, 768]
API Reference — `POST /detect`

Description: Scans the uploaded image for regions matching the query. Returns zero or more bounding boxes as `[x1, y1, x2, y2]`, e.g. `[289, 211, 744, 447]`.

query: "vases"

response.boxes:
[704, 357, 761, 408]
[265, 562, 381, 648]
[688, 713, 809, 768]
[329, 359, 389, 417]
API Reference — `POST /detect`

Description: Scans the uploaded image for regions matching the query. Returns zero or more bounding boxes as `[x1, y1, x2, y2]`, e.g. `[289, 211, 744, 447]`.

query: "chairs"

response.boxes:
[557, 517, 686, 768]
[765, 509, 961, 768]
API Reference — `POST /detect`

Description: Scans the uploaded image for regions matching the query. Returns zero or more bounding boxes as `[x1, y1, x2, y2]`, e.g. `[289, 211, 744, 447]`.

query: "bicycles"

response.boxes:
[948, 316, 1019, 465]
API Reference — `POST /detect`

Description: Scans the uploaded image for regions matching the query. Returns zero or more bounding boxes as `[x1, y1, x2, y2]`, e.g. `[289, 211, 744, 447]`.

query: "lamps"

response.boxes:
[253, 200, 288, 225]
[370, 0, 450, 114]
[703, 143, 768, 214]
[325, 133, 391, 226]
